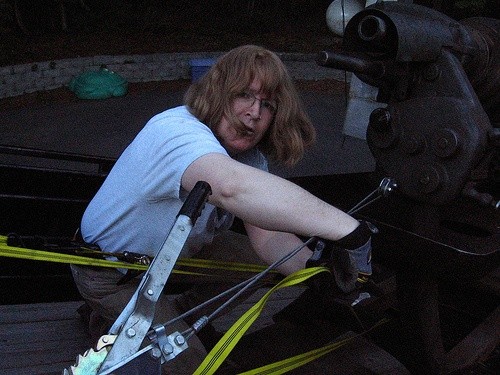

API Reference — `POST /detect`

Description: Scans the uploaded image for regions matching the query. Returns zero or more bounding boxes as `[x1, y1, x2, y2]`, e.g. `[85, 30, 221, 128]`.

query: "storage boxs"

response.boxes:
[191, 58, 215, 80]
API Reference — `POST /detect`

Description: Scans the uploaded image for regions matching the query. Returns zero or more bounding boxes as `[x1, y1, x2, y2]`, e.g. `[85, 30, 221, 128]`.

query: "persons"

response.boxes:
[70, 44, 374, 375]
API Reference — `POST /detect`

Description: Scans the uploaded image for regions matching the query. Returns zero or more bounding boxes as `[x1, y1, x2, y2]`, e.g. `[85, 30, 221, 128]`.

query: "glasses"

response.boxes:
[234, 90, 278, 114]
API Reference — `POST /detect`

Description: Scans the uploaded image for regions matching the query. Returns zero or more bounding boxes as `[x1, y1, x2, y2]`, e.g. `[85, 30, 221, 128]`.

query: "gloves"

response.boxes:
[305, 221, 379, 305]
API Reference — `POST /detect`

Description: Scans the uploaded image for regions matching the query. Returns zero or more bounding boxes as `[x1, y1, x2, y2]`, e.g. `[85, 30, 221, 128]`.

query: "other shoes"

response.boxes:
[76, 302, 92, 325]
